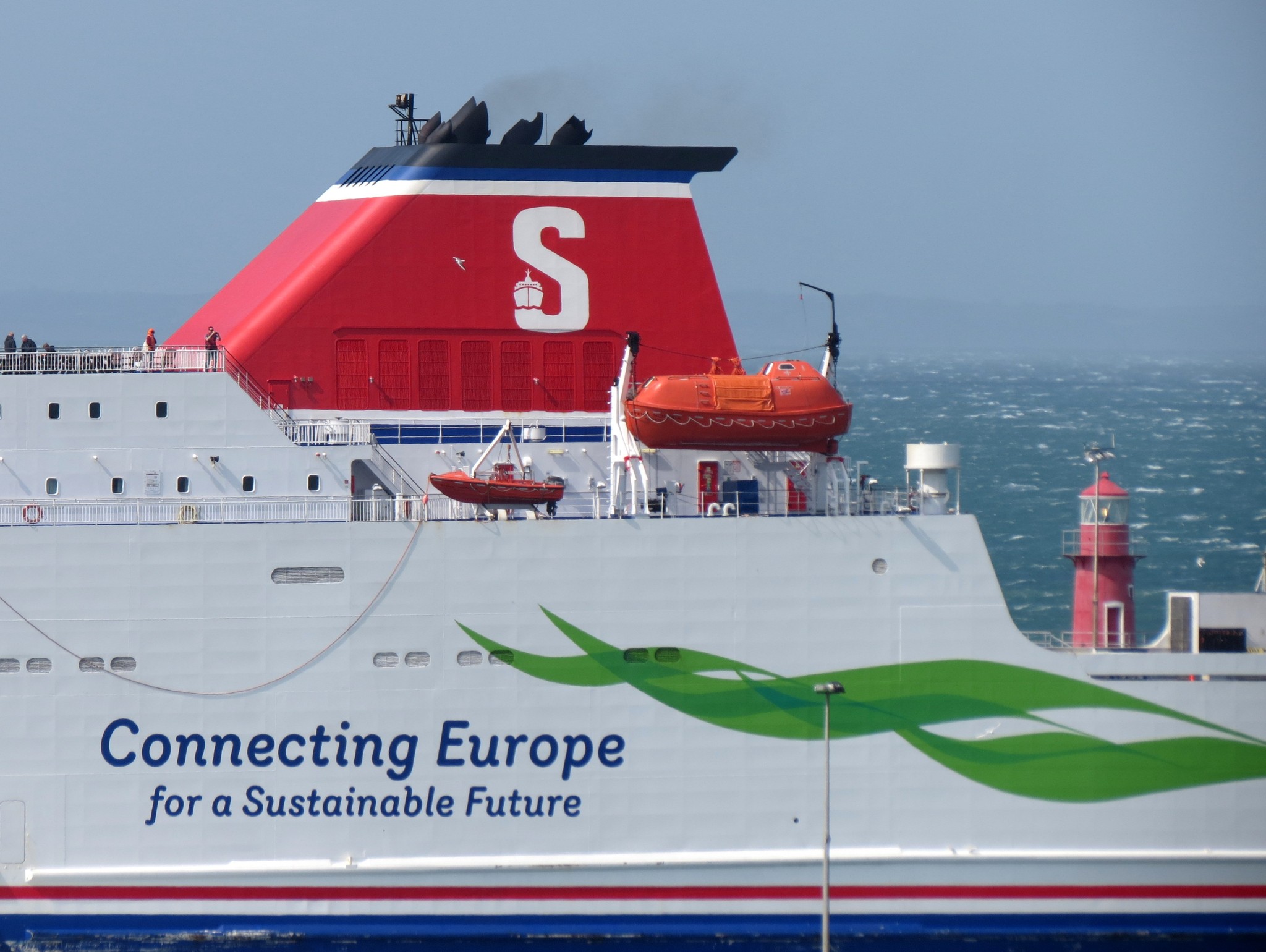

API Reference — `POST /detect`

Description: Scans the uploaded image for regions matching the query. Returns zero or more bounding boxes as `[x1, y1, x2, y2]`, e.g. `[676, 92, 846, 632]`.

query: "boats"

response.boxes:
[1, 90, 1266, 951]
[623, 358, 853, 459]
[422, 469, 566, 514]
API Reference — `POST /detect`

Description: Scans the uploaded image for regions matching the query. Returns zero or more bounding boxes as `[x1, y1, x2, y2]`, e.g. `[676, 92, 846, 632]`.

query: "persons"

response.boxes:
[204, 326, 221, 372]
[4, 333, 17, 362]
[42, 343, 58, 360]
[21, 334, 37, 354]
[146, 328, 157, 368]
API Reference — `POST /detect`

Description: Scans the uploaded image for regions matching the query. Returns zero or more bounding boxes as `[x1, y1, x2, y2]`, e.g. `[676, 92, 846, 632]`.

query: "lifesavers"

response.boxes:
[23, 503, 43, 523]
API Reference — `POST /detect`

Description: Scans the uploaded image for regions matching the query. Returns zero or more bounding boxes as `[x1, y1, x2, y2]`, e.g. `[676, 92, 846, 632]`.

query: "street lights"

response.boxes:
[815, 680, 846, 952]
